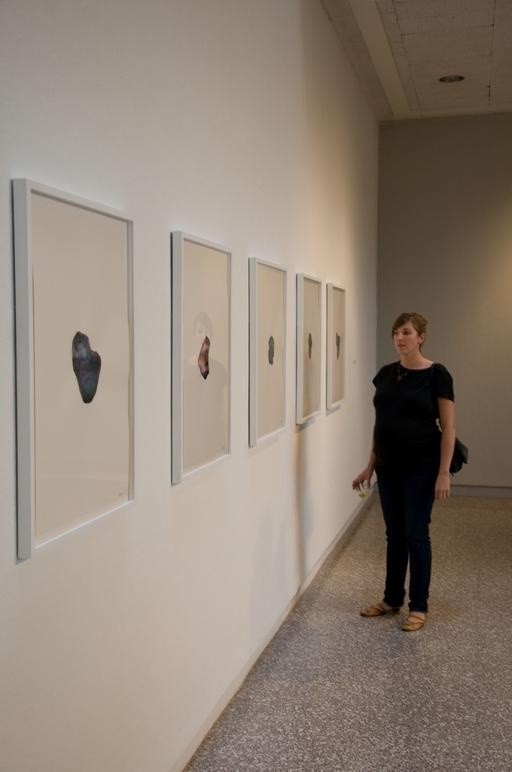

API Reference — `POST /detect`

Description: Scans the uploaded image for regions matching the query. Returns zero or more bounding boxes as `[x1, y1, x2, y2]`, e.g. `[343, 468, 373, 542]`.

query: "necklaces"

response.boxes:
[397, 365, 410, 381]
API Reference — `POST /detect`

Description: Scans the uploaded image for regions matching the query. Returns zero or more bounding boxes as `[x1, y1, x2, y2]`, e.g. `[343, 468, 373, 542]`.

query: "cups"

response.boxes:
[353, 482, 373, 500]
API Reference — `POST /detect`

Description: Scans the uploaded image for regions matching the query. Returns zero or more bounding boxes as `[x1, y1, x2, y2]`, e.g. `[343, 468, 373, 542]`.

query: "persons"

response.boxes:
[352, 312, 457, 631]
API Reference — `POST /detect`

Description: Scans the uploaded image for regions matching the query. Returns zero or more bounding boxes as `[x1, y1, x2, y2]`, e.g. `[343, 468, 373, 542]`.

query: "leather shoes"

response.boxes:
[360, 602, 400, 617]
[400, 611, 427, 631]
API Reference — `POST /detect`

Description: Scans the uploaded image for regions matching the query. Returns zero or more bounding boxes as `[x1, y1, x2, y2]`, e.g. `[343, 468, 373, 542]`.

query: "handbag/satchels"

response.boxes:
[450, 438, 468, 472]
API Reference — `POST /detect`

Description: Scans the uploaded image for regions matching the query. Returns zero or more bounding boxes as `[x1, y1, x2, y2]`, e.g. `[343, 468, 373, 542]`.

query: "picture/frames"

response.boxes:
[13, 179, 145, 559]
[249, 257, 287, 447]
[296, 273, 324, 428]
[326, 282, 346, 413]
[172, 232, 235, 484]
